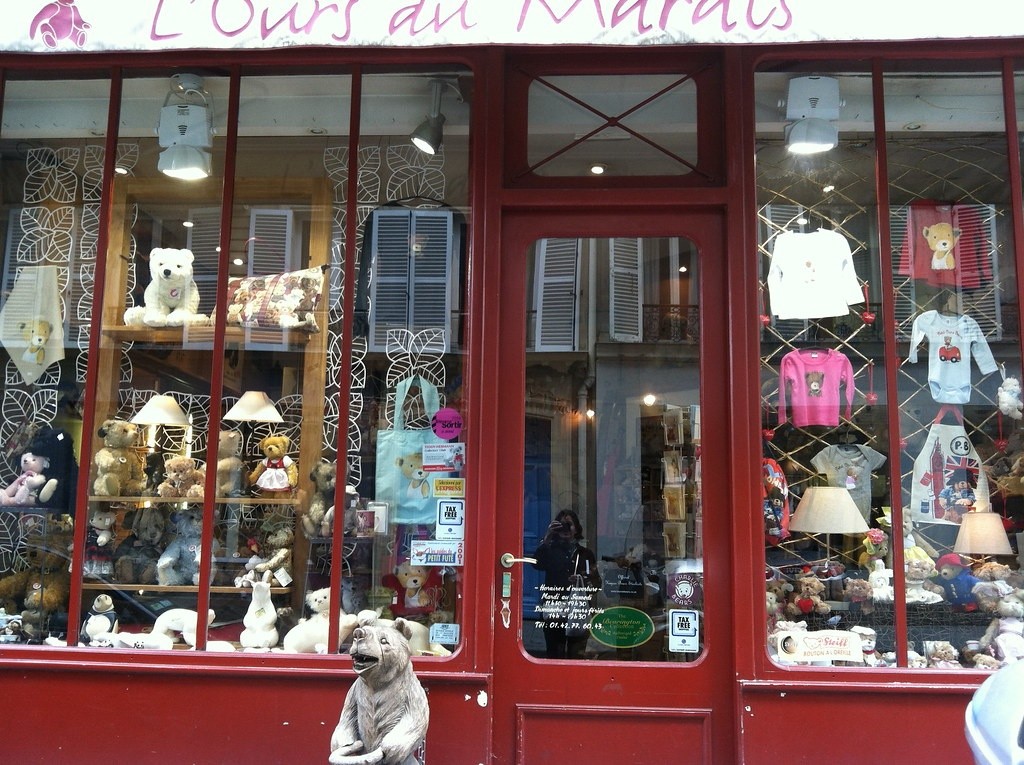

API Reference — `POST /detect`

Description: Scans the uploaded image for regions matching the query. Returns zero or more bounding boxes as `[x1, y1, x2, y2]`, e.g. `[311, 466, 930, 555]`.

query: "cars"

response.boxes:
[0, 528, 246, 644]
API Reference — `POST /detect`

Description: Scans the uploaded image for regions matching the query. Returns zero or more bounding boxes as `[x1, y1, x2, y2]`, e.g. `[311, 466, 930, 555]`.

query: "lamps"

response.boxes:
[953, 513, 1013, 567]
[158, 73, 210, 182]
[790, 486, 871, 561]
[410, 74, 454, 156]
[782, 74, 845, 155]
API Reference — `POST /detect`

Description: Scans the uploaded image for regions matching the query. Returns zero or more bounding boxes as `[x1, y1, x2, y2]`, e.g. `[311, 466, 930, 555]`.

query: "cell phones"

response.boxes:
[554, 523, 571, 532]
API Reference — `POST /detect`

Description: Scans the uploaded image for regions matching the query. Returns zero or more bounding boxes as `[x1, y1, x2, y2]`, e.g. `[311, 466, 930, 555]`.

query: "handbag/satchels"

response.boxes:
[565, 574, 593, 638]
[208, 237, 332, 333]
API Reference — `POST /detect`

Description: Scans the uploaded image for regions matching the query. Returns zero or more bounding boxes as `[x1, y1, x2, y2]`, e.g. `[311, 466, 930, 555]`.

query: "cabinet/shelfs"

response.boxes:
[81, 176, 332, 624]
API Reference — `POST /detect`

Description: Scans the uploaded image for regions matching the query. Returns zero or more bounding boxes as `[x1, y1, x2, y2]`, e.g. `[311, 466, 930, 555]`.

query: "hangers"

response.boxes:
[766, 178, 984, 468]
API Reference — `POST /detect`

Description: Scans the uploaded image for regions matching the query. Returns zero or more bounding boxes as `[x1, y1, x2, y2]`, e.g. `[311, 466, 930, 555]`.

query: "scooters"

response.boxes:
[600, 543, 705, 664]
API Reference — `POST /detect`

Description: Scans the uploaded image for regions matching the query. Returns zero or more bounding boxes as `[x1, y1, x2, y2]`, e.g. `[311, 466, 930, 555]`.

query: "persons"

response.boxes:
[533, 511, 601, 659]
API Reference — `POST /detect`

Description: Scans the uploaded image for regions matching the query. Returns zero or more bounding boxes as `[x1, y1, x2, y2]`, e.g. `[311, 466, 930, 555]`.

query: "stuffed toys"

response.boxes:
[765, 508, 1023, 670]
[0, 533, 70, 640]
[284, 577, 452, 656]
[250, 437, 298, 499]
[198, 431, 242, 498]
[124, 248, 213, 327]
[67, 505, 294, 587]
[157, 457, 205, 497]
[302, 460, 362, 536]
[998, 378, 1024, 420]
[0, 427, 79, 507]
[94, 420, 149, 496]
